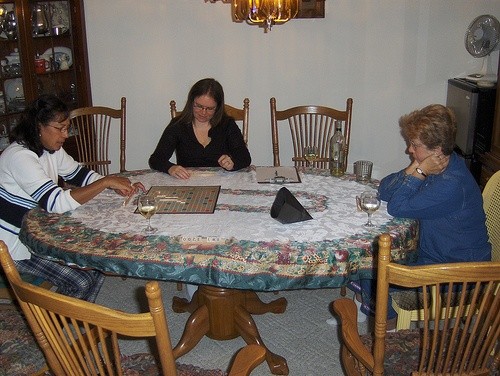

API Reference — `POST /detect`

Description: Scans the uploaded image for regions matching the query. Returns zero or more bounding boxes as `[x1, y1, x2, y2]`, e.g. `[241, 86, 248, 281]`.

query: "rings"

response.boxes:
[438, 155, 442, 160]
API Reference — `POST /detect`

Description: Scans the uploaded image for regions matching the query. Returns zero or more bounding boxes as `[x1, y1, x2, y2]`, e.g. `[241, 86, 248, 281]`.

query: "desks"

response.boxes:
[18, 164, 420, 376]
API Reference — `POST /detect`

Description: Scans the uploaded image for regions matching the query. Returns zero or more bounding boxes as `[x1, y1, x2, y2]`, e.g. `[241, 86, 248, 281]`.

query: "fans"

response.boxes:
[466, 13, 500, 83]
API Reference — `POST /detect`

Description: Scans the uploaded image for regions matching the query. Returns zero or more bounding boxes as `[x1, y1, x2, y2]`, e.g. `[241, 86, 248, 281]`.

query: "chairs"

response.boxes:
[0, 241, 225, 376]
[170, 98, 249, 147]
[333, 232, 500, 376]
[57, 97, 127, 189]
[391, 170, 500, 329]
[270, 98, 353, 172]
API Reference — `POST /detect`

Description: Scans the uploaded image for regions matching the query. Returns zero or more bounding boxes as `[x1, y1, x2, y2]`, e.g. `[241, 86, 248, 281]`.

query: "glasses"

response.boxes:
[192, 103, 216, 112]
[407, 140, 425, 151]
[46, 124, 73, 133]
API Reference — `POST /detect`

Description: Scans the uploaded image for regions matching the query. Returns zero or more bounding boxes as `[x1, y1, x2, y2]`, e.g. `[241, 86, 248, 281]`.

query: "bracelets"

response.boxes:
[403, 168, 410, 175]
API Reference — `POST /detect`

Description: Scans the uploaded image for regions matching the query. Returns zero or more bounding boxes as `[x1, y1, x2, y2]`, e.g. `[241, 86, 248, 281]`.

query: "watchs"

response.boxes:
[415, 167, 427, 178]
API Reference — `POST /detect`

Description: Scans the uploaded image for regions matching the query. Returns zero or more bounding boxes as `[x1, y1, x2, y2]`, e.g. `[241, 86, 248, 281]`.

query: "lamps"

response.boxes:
[222, 0, 298, 32]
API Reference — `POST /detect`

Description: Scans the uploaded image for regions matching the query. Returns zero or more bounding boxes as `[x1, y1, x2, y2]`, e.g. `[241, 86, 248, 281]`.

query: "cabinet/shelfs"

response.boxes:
[0, 0, 98, 186]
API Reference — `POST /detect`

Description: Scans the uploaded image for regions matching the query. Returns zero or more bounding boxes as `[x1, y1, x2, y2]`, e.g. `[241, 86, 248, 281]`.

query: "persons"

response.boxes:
[149, 77, 252, 180]
[0, 95, 147, 332]
[325, 104, 491, 334]
